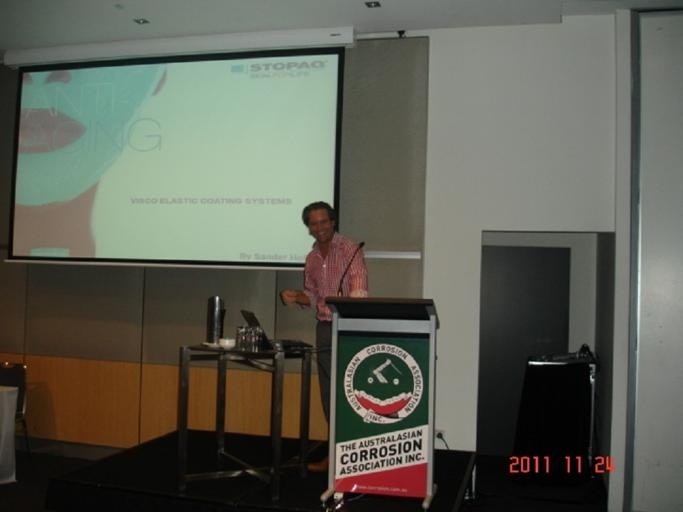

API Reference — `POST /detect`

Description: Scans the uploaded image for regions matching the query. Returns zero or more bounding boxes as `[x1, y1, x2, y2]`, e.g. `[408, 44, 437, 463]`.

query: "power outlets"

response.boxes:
[434, 428, 446, 439]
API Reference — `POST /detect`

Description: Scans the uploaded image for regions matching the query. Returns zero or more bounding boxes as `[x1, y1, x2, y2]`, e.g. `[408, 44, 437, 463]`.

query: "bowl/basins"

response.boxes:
[220, 338, 236, 350]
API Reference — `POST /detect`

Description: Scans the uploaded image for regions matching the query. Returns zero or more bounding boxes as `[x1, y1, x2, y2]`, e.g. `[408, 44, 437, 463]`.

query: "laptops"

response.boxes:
[240, 309, 313, 350]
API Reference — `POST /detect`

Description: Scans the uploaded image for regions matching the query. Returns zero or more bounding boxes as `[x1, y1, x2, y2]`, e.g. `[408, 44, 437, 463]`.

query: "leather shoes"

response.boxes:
[306, 456, 329, 472]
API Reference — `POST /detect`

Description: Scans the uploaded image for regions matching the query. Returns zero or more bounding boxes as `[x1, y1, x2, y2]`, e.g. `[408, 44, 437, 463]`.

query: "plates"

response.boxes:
[208, 344, 221, 348]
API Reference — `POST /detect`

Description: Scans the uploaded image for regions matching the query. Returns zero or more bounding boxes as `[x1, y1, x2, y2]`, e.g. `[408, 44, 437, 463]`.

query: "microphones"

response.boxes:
[337, 241, 366, 296]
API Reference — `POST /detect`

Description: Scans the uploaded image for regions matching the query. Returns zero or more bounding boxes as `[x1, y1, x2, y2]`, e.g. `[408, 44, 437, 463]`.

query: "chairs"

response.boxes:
[0, 362, 31, 453]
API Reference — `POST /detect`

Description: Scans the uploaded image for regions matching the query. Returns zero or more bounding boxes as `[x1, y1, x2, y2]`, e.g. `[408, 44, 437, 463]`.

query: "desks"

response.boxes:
[176, 339, 313, 505]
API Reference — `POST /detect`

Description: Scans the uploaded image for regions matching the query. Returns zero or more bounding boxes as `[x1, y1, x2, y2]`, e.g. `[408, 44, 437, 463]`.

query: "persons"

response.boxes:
[281, 201, 369, 470]
